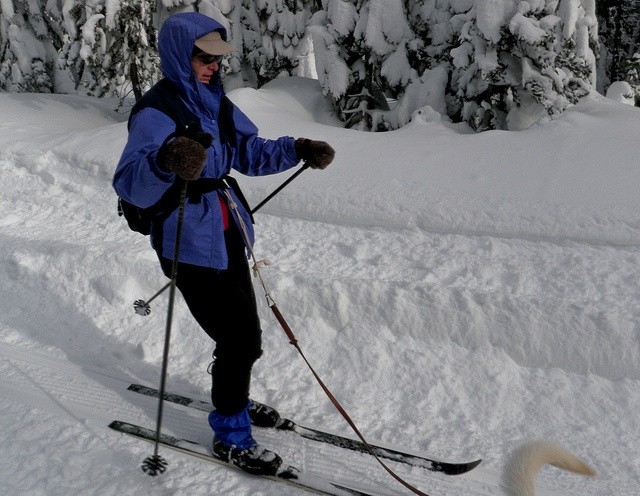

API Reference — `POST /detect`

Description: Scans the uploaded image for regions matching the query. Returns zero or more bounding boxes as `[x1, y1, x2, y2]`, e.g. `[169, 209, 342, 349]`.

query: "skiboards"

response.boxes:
[108, 383, 483, 495]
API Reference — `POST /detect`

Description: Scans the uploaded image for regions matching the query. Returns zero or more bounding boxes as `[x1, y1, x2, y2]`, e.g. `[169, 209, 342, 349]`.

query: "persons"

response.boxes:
[112, 13, 336, 476]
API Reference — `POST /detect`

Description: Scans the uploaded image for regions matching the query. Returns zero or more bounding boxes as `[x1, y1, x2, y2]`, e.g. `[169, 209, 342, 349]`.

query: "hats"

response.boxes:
[194, 32, 239, 55]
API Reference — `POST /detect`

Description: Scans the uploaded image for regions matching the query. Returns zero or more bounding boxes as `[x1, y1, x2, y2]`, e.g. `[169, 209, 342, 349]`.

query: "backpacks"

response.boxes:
[117, 79, 237, 235]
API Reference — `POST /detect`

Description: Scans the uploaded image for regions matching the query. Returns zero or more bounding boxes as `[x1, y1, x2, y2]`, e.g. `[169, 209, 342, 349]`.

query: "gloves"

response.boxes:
[156, 136, 210, 181]
[293, 138, 335, 170]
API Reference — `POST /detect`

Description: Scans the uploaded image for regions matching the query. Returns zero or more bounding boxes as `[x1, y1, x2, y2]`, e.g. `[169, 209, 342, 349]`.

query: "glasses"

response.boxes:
[195, 55, 223, 64]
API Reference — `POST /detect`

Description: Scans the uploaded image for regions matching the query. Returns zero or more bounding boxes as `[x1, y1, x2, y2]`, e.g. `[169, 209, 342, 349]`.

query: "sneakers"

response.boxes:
[213, 434, 283, 475]
[247, 398, 279, 428]
